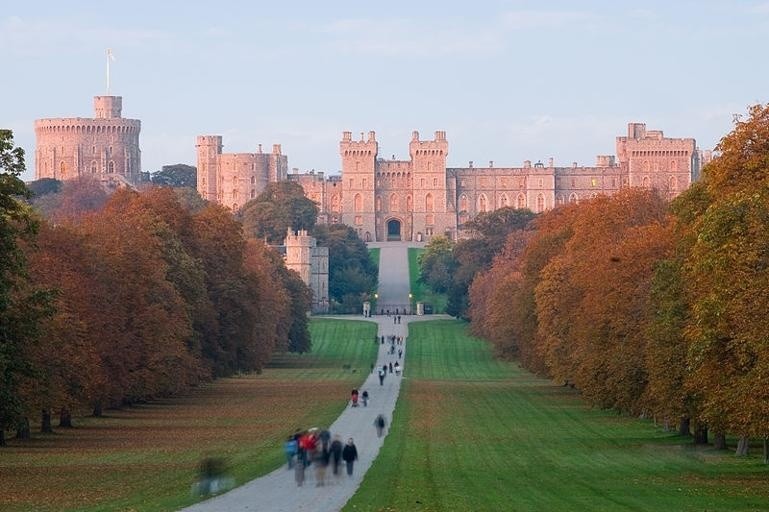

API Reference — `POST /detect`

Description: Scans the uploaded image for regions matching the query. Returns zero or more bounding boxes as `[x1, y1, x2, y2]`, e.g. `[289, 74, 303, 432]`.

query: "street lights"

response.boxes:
[374, 294, 378, 315]
[408, 293, 414, 315]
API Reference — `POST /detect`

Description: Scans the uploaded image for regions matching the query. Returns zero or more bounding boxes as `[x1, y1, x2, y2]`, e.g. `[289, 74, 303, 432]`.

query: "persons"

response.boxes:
[348, 388, 369, 407]
[376, 308, 409, 324]
[372, 334, 404, 359]
[371, 361, 402, 386]
[364, 309, 372, 319]
[286, 428, 358, 488]
[373, 413, 387, 437]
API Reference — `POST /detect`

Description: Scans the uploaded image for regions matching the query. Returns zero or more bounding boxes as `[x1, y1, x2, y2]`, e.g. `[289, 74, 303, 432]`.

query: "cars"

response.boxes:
[425, 304, 433, 313]
